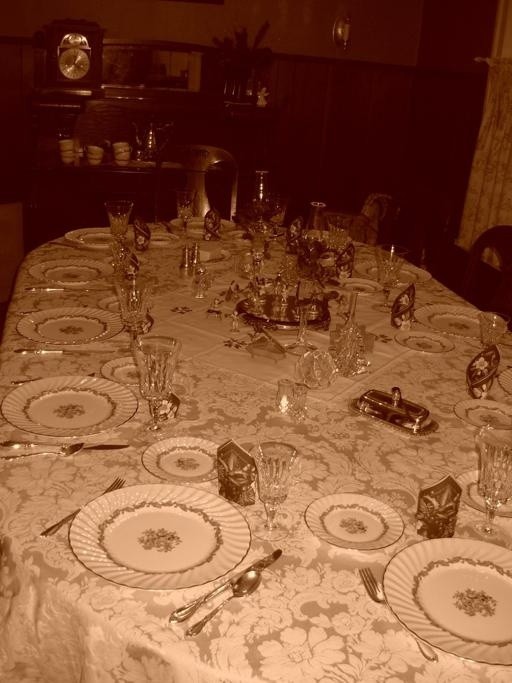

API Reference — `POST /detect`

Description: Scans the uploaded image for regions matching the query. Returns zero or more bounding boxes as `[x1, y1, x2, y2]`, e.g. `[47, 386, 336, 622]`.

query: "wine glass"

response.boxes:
[371, 239, 508, 347]
[247, 440, 300, 541]
[471, 423, 512, 541]
[174, 191, 196, 234]
[330, 283, 373, 378]
[102, 198, 180, 441]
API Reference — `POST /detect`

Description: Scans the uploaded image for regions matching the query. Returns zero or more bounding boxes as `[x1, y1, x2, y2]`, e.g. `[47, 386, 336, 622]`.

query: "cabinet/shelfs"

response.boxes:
[17, 38, 277, 253]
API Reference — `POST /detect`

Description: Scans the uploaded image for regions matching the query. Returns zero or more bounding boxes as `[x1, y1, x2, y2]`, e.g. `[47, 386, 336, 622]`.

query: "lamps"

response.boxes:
[331, 14, 352, 49]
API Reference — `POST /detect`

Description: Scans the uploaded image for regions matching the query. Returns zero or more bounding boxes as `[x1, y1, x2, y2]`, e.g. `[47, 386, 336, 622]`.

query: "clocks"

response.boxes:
[35, 17, 106, 92]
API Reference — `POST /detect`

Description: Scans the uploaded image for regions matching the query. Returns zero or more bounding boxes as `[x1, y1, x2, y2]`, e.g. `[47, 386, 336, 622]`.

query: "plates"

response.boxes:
[330, 256, 507, 355]
[305, 491, 405, 553]
[453, 399, 511, 434]
[383, 538, 511, 666]
[1, 226, 142, 438]
[144, 212, 259, 246]
[451, 467, 512, 517]
[69, 482, 252, 592]
[498, 366, 511, 395]
[140, 434, 223, 484]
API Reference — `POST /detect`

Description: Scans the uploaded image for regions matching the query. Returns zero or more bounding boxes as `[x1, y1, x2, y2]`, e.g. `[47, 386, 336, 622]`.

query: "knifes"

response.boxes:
[1, 441, 130, 451]
[169, 550, 280, 624]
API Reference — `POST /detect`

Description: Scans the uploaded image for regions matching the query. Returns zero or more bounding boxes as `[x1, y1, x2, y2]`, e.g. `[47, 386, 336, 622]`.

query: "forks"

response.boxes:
[358, 564, 438, 665]
[38, 476, 130, 538]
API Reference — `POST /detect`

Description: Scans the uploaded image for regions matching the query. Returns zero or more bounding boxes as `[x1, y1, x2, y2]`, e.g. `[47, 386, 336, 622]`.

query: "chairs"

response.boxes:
[466, 220, 512, 328]
[151, 143, 239, 226]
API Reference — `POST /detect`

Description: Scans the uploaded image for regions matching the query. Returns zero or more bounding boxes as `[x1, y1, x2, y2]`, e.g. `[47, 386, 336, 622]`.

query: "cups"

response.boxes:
[306, 200, 350, 253]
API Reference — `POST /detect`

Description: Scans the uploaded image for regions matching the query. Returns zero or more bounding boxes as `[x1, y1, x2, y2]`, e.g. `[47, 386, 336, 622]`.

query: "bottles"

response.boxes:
[276, 379, 308, 421]
[182, 241, 200, 270]
[249, 169, 277, 235]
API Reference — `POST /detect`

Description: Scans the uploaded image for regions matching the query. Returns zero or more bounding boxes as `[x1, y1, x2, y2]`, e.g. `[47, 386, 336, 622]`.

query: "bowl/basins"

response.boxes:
[58, 136, 133, 165]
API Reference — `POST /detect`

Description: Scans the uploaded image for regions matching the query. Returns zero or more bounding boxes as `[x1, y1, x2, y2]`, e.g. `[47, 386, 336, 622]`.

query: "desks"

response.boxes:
[0, 186, 512, 682]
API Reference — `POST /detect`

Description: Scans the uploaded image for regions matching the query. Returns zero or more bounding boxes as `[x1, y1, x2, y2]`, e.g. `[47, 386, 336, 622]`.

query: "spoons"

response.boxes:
[187, 566, 262, 639]
[2, 441, 85, 463]
